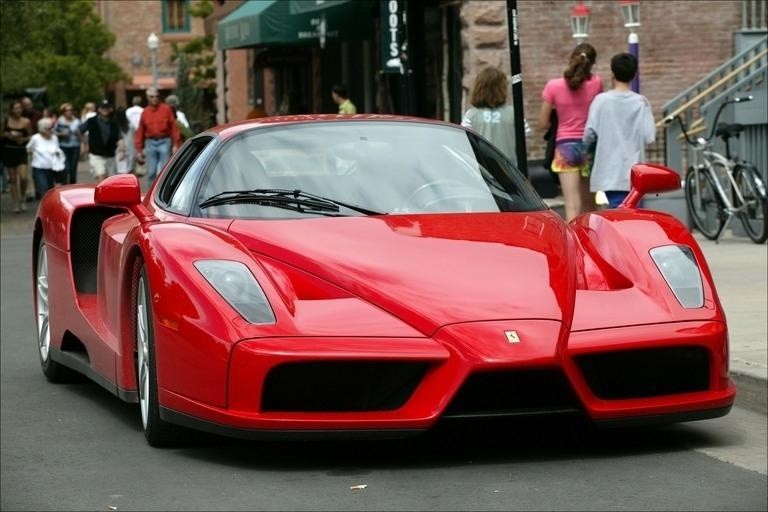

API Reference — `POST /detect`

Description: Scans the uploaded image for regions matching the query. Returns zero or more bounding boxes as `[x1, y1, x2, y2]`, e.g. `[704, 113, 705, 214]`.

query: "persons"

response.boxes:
[460, 67, 532, 169]
[244, 97, 268, 118]
[333, 84, 356, 115]
[2, 86, 190, 212]
[538, 42, 606, 223]
[582, 53, 658, 210]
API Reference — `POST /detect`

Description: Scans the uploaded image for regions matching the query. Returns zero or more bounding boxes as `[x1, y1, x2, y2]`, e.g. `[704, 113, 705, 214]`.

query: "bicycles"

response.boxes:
[663, 95, 767, 243]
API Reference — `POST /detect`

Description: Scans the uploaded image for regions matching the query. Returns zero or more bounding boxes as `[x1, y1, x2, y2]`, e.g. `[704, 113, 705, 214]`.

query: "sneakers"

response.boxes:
[12, 192, 34, 213]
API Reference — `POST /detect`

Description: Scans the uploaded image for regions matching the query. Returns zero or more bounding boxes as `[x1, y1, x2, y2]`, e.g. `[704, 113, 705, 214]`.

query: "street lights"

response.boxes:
[143, 33, 162, 91]
[566, 0, 590, 59]
[618, 0, 641, 99]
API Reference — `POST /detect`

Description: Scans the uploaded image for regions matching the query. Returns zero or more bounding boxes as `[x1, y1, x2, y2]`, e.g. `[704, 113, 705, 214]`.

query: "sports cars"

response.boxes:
[29, 110, 737, 449]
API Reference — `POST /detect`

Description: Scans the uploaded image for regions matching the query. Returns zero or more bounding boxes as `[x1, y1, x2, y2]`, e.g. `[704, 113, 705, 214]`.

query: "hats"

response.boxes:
[98, 99, 110, 108]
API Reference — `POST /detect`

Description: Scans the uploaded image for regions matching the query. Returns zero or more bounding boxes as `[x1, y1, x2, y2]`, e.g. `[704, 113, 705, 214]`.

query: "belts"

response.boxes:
[149, 135, 169, 140]
